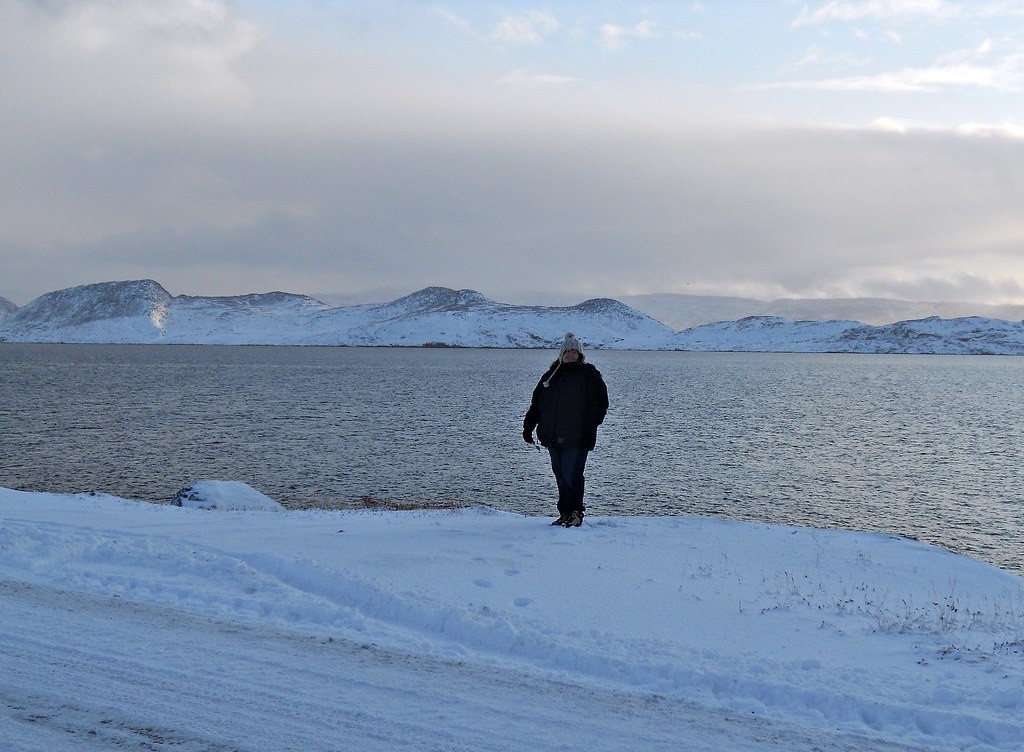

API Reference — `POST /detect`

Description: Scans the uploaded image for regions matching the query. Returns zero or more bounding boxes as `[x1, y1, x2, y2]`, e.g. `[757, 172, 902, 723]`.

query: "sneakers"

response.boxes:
[550, 509, 585, 528]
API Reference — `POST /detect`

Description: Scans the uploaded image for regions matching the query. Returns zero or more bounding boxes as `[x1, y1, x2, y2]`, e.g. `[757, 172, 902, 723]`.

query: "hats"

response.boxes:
[542, 331, 587, 388]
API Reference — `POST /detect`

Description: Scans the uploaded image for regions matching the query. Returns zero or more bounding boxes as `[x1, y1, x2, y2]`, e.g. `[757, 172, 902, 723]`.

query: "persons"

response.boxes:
[522, 332, 610, 528]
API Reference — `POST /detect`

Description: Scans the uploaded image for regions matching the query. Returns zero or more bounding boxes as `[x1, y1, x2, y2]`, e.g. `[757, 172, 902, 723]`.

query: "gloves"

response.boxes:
[522, 430, 533, 444]
